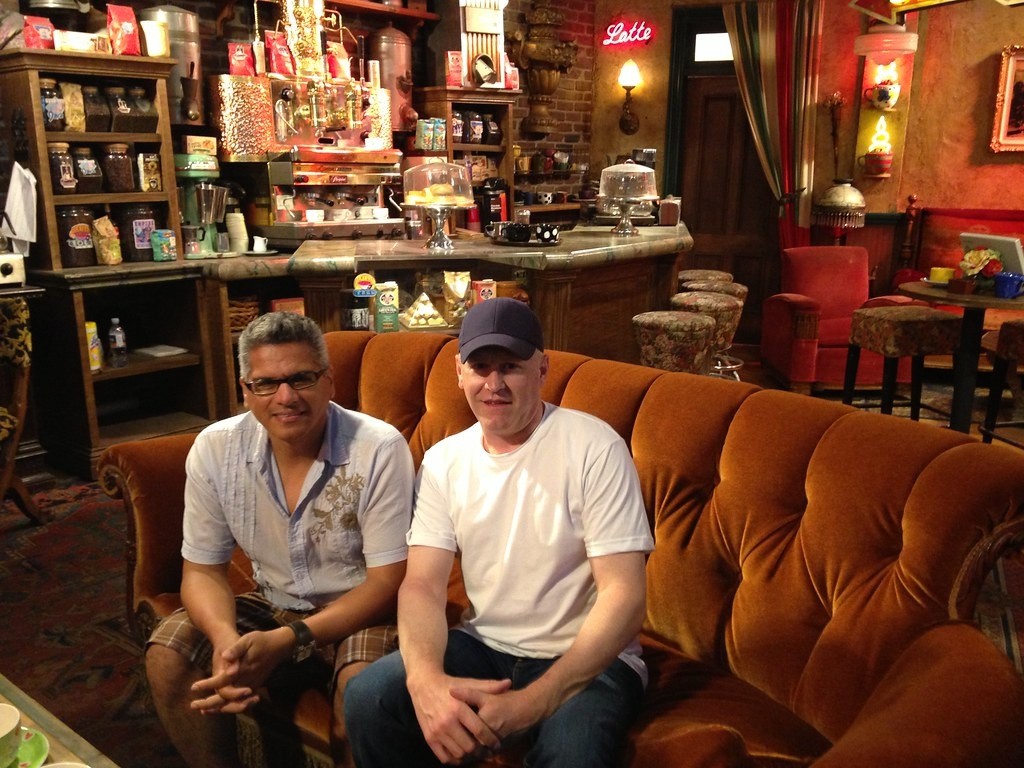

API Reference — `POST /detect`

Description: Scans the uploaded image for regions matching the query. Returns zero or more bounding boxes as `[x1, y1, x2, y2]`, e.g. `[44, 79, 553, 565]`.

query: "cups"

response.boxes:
[863, 84, 900, 110]
[857, 152, 893, 176]
[41, 761, 92, 768]
[0, 704, 22, 768]
[194, 182, 230, 226]
[485, 221, 560, 242]
[996, 271, 1024, 299]
[512, 145, 595, 207]
[929, 267, 956, 281]
[215, 212, 269, 253]
[306, 207, 390, 221]
[949, 280, 976, 294]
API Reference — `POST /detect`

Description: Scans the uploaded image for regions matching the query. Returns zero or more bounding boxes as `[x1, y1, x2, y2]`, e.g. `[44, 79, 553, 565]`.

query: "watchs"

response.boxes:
[285, 621, 315, 666]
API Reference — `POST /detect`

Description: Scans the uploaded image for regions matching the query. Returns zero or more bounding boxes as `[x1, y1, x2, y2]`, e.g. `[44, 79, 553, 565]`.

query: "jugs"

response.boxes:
[181, 221, 207, 243]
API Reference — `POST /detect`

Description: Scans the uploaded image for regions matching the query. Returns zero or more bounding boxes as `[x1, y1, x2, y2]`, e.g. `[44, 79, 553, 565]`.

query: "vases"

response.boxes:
[864, 86, 901, 109]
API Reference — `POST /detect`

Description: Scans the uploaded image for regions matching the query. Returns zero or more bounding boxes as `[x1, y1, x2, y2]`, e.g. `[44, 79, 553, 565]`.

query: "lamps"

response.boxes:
[810, 177, 867, 245]
[852, 24, 919, 65]
[617, 59, 641, 136]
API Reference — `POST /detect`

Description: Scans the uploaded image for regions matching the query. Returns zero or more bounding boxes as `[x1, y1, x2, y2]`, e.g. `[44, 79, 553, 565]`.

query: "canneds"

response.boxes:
[38, 78, 159, 267]
[452, 107, 502, 144]
[406, 180, 471, 206]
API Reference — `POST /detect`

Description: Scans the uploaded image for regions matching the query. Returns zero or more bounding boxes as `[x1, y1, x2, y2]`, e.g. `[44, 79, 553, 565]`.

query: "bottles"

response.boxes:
[85, 317, 129, 372]
[39, 79, 159, 267]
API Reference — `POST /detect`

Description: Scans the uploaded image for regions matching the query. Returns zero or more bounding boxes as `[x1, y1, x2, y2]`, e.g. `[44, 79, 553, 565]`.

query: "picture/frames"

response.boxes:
[989, 44, 1024, 155]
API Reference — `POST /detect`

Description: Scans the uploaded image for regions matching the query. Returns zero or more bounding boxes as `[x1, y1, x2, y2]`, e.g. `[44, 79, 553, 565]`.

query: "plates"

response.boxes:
[219, 250, 278, 257]
[919, 277, 949, 287]
[9, 726, 50, 768]
[489, 237, 560, 246]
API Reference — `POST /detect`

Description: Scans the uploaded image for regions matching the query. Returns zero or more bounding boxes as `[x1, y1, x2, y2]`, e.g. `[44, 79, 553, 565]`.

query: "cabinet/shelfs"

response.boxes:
[413, 85, 523, 233]
[863, 108, 897, 178]
[0, 47, 184, 271]
[26, 261, 217, 485]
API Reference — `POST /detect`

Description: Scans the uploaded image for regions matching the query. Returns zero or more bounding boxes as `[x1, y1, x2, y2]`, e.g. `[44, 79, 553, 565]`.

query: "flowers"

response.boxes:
[878, 80, 899, 87]
[822, 91, 847, 171]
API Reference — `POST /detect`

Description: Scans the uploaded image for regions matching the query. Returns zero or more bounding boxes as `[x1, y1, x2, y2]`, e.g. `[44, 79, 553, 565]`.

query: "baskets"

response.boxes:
[229, 299, 258, 332]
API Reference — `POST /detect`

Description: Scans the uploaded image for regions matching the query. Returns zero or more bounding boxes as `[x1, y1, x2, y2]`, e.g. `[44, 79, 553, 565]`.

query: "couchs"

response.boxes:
[759, 244, 914, 403]
[98, 328, 1024, 768]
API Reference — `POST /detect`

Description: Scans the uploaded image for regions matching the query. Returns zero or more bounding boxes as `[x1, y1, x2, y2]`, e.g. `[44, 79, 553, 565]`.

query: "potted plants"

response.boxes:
[857, 148, 894, 174]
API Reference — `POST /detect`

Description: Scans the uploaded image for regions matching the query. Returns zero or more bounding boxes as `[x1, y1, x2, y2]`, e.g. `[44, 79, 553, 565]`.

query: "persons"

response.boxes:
[144, 307, 417, 768]
[342, 298, 655, 768]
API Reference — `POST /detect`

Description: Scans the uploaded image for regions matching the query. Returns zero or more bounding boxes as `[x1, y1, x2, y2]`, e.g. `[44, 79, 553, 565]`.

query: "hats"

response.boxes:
[458, 298, 544, 364]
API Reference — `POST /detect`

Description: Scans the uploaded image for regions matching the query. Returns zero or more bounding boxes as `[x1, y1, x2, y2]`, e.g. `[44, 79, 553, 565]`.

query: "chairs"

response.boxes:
[0, 297, 56, 527]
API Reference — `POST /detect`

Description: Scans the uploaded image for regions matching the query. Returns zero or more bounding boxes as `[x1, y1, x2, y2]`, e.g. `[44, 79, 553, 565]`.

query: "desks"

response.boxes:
[899, 281, 1024, 435]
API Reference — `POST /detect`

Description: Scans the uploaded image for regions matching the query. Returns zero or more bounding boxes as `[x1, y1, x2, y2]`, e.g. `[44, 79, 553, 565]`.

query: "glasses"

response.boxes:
[243, 368, 327, 396]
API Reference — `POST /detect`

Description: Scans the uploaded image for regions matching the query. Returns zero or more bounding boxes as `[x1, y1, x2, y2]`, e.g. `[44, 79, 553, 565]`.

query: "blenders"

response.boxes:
[174, 153, 241, 258]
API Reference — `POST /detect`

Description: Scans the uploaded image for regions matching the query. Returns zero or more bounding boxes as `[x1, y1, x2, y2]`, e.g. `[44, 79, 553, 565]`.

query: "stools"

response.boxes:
[841, 305, 961, 422]
[672, 292, 744, 382]
[632, 312, 716, 377]
[677, 270, 735, 292]
[681, 282, 749, 358]
[978, 318, 1024, 452]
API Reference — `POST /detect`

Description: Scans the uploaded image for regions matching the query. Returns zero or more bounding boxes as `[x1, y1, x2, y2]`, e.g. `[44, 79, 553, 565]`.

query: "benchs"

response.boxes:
[890, 196, 1024, 384]
